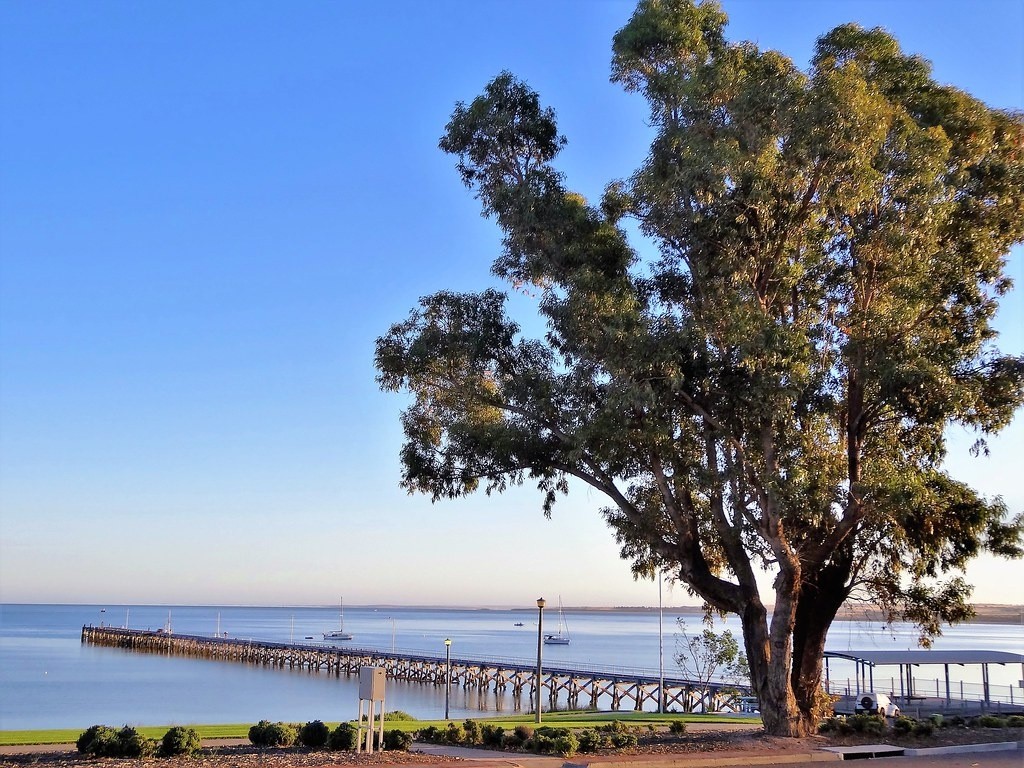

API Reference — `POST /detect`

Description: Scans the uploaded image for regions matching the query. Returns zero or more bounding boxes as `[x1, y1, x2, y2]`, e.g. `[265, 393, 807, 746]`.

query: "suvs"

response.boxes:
[854, 693, 900, 718]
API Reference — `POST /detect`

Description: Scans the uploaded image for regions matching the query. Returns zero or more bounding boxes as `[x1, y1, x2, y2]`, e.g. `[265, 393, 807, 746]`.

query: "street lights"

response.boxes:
[444, 637, 451, 719]
[535, 598, 546, 724]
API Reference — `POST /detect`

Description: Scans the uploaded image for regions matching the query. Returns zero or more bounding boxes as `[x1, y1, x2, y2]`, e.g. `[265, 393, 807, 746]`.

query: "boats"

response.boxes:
[305, 637, 313, 639]
[101, 611, 105, 612]
[514, 623, 525, 626]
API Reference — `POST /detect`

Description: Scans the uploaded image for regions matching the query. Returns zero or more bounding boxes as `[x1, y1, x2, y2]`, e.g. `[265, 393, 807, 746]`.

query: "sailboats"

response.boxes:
[323, 595, 353, 640]
[544, 595, 572, 645]
